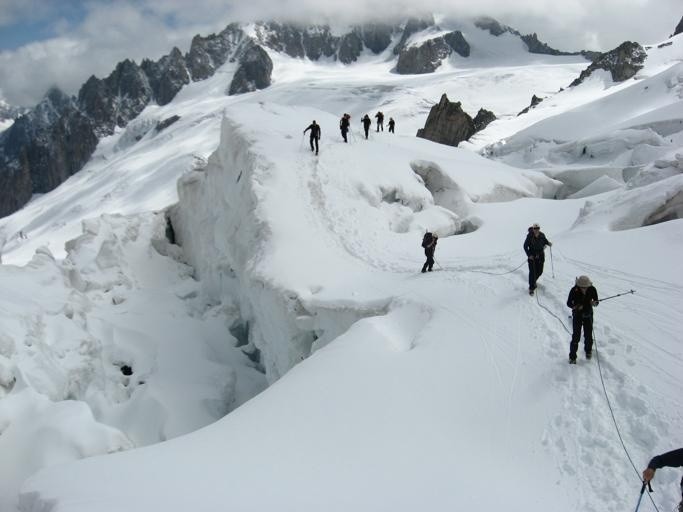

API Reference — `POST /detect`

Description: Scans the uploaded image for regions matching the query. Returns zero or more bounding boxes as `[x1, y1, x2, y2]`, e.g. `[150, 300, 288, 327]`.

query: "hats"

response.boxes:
[533, 223, 540, 229]
[576, 276, 593, 287]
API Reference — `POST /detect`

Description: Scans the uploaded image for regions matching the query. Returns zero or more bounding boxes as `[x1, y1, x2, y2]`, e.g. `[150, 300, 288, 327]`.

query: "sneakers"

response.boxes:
[569, 358, 576, 364]
[529, 283, 537, 296]
[586, 353, 592, 360]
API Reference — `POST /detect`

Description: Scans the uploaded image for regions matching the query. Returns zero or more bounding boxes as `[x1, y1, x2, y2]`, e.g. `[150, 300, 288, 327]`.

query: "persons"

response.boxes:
[641, 447, 682, 511]
[373, 111, 384, 132]
[420, 231, 439, 273]
[302, 119, 321, 156]
[360, 114, 372, 140]
[565, 274, 600, 364]
[338, 113, 352, 144]
[522, 223, 552, 296]
[385, 117, 396, 134]
